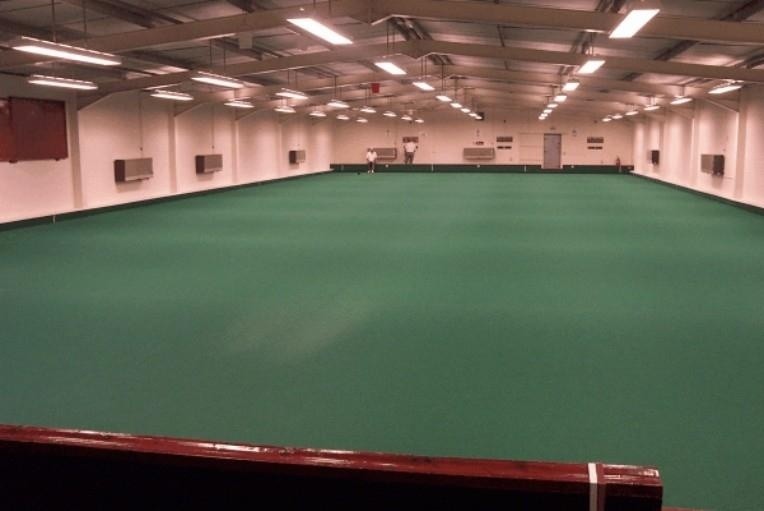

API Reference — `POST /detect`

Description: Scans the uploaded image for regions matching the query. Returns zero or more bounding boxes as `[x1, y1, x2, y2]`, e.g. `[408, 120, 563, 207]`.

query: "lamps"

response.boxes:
[27, 59, 98, 91]
[311, 75, 424, 126]
[189, 38, 243, 89]
[1, 1, 123, 68]
[272, 96, 297, 114]
[412, 48, 483, 127]
[222, 89, 255, 109]
[284, 1, 353, 50]
[537, 0, 750, 125]
[373, 19, 407, 78]
[277, 69, 308, 101]
[149, 84, 194, 102]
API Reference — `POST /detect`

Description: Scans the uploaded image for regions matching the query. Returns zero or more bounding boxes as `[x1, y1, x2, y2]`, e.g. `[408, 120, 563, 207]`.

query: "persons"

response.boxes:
[365, 147, 378, 174]
[614, 155, 621, 173]
[402, 138, 418, 165]
